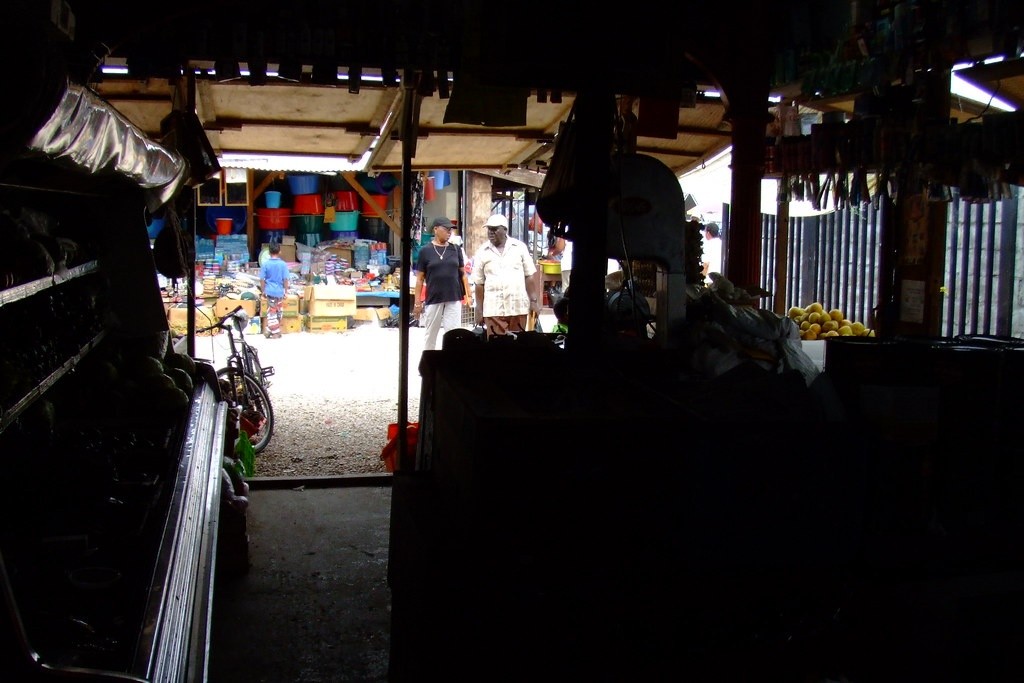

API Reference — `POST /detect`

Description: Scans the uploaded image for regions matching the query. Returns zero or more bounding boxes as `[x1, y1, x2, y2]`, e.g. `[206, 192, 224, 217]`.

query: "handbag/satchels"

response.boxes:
[159, 86, 222, 189]
[536, 92, 596, 225]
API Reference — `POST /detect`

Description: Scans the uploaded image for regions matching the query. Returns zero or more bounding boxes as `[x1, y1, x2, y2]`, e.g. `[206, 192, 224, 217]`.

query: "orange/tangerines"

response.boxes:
[786, 302, 874, 340]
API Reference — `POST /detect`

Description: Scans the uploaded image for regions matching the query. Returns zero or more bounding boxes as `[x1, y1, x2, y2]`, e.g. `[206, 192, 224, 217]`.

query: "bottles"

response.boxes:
[234, 431, 254, 476]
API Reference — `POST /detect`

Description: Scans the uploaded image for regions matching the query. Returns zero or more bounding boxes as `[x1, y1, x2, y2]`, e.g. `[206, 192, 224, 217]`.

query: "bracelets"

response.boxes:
[414, 303, 421, 308]
[531, 298, 537, 303]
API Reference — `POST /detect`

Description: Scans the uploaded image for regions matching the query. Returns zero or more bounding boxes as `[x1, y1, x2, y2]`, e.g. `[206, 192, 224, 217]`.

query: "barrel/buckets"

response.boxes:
[215, 218, 233, 235]
[362, 217, 389, 243]
[429, 171, 444, 191]
[354, 249, 370, 270]
[370, 250, 387, 265]
[259, 174, 391, 246]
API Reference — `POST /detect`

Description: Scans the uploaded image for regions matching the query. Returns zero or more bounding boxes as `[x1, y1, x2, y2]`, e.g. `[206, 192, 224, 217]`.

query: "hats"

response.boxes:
[431, 216, 458, 229]
[482, 214, 508, 229]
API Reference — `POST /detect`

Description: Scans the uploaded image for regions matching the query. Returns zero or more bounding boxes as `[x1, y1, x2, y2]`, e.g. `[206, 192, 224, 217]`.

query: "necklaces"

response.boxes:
[432, 241, 447, 259]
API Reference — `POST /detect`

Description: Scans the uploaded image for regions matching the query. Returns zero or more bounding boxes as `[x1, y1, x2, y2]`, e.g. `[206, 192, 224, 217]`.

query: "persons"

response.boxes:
[413, 217, 472, 350]
[475, 214, 540, 335]
[551, 237, 573, 293]
[260, 242, 289, 338]
[701, 224, 722, 284]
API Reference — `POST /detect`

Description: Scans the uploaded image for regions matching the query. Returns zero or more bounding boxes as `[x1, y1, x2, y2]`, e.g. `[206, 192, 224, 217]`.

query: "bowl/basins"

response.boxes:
[541, 263, 562, 274]
[205, 206, 247, 234]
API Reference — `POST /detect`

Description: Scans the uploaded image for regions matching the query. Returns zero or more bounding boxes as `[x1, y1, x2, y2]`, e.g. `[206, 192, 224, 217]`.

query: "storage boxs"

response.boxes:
[160, 236, 392, 333]
[540, 261, 562, 274]
[616, 94, 680, 139]
[442, 69, 527, 126]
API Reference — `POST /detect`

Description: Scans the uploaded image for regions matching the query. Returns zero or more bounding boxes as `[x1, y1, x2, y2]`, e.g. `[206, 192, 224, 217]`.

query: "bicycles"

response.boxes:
[195, 305, 276, 455]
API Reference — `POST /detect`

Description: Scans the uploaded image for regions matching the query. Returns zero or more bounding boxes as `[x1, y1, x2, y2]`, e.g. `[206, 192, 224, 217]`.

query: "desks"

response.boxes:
[539, 265, 562, 306]
[355, 291, 400, 308]
